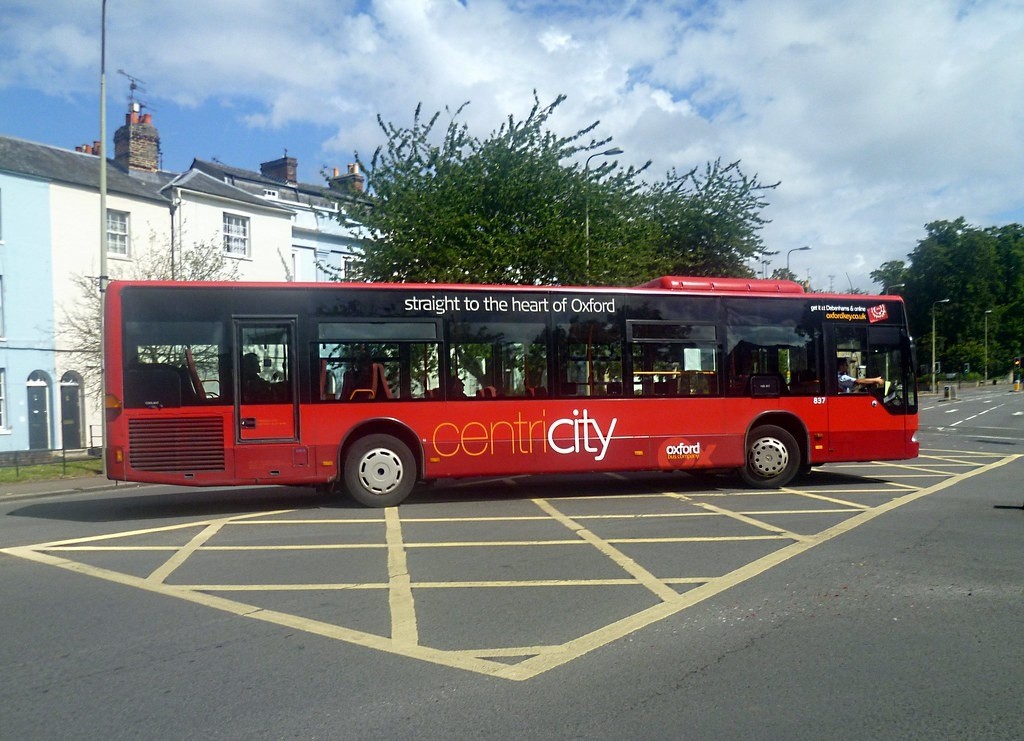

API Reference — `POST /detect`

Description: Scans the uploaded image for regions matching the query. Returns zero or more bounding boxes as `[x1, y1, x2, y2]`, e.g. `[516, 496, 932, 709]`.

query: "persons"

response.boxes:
[836, 357, 884, 392]
[241, 353, 278, 405]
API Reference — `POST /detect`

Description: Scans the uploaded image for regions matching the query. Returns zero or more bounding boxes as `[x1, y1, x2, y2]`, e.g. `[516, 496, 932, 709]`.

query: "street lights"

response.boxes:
[886, 283, 905, 295]
[585, 147, 623, 286]
[787, 246, 811, 279]
[985, 310, 992, 385]
[932, 298, 950, 391]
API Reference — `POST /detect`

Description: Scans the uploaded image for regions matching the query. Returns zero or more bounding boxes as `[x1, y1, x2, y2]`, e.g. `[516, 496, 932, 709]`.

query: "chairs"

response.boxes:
[184, 349, 678, 399]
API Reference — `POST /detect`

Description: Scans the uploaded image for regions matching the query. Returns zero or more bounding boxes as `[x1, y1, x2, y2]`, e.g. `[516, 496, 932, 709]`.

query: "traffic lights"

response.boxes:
[964, 363, 969, 373]
[1014, 358, 1020, 370]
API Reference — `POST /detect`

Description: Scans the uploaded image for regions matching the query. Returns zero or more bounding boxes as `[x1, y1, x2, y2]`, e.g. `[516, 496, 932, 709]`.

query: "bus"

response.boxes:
[100, 275, 918, 510]
